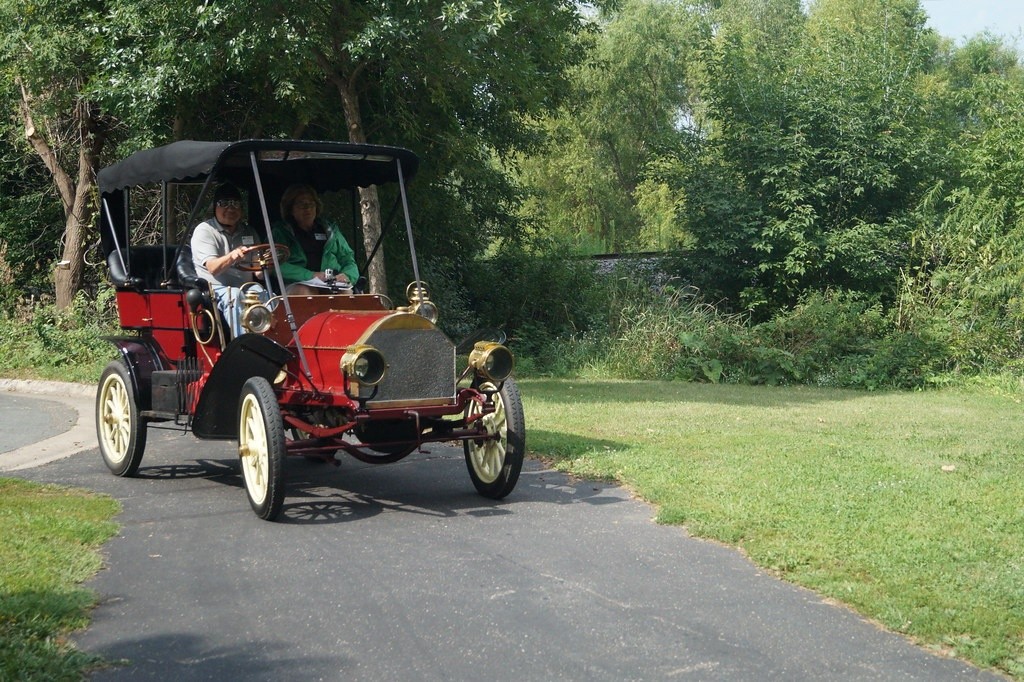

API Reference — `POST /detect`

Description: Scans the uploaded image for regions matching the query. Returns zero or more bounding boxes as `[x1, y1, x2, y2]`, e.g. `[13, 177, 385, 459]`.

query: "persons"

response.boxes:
[190, 184, 279, 339]
[263, 184, 360, 295]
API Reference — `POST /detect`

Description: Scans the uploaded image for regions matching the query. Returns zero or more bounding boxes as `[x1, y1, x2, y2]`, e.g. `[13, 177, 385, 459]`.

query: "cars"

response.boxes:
[94, 139, 526, 521]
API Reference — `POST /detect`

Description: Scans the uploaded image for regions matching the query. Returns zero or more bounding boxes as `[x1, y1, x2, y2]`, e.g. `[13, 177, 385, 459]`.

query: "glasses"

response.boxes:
[216, 200, 244, 209]
[293, 202, 316, 209]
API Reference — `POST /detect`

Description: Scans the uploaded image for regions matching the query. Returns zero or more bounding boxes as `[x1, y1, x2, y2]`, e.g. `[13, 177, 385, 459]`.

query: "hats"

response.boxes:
[213, 183, 242, 217]
[280, 184, 321, 221]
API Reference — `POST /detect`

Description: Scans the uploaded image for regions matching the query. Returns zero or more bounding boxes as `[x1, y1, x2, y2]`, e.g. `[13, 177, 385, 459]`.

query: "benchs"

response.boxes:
[108, 244, 366, 333]
